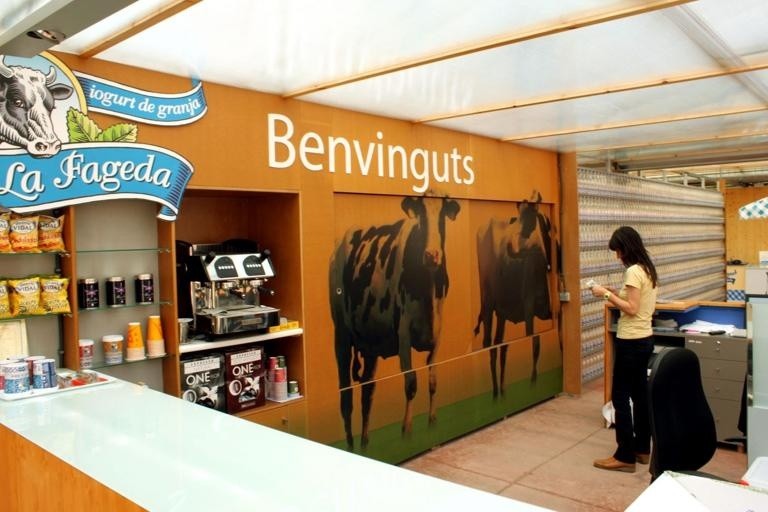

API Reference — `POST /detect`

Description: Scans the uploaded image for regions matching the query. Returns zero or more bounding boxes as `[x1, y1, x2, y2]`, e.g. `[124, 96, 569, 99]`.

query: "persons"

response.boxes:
[586, 225, 657, 473]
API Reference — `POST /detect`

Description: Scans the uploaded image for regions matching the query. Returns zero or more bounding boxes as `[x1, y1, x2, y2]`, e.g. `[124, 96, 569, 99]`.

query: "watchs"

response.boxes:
[604, 291, 610, 302]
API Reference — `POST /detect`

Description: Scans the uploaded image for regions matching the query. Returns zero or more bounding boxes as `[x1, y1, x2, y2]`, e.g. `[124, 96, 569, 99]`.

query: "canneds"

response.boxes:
[80, 278, 100, 311]
[135, 272, 154, 305]
[105, 275, 126, 309]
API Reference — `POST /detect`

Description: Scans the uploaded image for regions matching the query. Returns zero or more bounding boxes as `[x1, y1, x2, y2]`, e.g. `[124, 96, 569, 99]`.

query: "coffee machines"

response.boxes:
[177, 240, 279, 334]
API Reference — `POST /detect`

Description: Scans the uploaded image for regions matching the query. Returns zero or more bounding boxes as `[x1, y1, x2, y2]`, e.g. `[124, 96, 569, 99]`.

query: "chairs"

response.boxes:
[634, 342, 746, 482]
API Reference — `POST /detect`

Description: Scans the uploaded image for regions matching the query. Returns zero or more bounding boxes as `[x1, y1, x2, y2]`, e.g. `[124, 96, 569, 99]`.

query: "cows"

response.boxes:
[473, 191, 565, 400]
[329, 190, 461, 452]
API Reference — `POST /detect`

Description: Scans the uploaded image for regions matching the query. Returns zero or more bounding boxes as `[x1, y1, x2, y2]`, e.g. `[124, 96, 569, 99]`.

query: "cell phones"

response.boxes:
[710, 330, 726, 335]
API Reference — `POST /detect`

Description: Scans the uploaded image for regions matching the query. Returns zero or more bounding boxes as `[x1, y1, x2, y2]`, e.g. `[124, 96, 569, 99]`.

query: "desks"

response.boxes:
[613, 455, 768, 512]
[602, 292, 753, 452]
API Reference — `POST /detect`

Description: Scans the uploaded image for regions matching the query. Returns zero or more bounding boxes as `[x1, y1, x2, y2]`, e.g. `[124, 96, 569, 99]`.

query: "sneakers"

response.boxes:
[592, 456, 636, 473]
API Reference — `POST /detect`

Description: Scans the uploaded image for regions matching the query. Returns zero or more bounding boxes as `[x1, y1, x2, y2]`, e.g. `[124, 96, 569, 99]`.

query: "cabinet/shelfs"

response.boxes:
[743, 295, 768, 468]
[0, 241, 69, 321]
[73, 246, 170, 369]
[238, 398, 310, 439]
[172, 181, 308, 415]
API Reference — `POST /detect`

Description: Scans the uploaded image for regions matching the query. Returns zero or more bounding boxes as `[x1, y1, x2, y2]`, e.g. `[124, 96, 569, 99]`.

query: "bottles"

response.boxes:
[79, 338, 94, 367]
[0, 355, 58, 398]
[267, 355, 302, 398]
[103, 333, 123, 366]
[77, 272, 155, 308]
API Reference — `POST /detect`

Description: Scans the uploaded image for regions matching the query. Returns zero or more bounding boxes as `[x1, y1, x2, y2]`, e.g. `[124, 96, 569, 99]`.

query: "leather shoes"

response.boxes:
[636, 453, 650, 464]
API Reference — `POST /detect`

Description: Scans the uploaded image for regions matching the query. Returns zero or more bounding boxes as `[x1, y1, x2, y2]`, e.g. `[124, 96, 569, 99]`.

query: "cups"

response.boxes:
[126, 315, 168, 361]
[177, 318, 195, 344]
